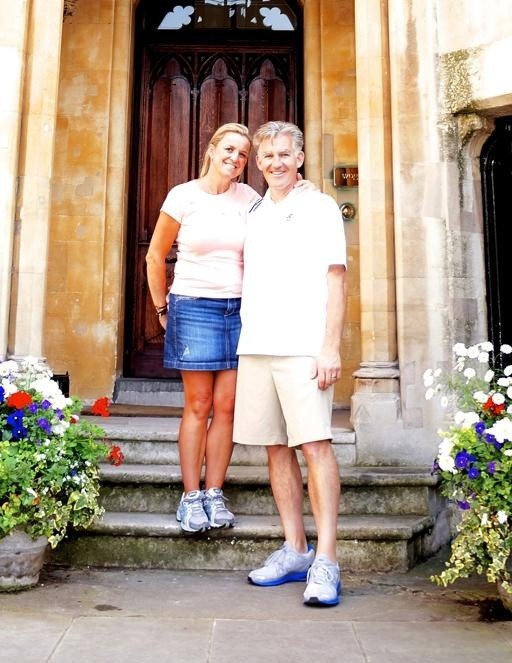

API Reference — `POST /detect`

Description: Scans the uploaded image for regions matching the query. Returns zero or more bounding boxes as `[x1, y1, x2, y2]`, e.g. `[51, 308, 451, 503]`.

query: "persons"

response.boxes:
[144, 122, 319, 539]
[231, 119, 349, 609]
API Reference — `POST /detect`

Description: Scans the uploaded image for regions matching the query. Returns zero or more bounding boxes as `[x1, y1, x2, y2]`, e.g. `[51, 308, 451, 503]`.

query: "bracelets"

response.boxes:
[154, 304, 167, 311]
[156, 309, 168, 318]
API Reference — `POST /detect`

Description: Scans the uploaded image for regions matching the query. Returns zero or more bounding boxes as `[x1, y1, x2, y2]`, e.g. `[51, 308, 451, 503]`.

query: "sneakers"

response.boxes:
[303, 555, 341, 606]
[247, 540, 316, 586]
[177, 487, 208, 532]
[203, 487, 235, 529]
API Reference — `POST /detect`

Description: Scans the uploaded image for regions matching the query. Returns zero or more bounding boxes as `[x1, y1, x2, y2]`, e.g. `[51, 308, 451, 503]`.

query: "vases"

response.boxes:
[494, 554, 511, 614]
[1, 519, 50, 592]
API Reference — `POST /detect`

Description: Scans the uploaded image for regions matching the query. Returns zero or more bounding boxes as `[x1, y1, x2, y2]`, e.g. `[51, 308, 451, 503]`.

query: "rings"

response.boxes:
[331, 376, 337, 378]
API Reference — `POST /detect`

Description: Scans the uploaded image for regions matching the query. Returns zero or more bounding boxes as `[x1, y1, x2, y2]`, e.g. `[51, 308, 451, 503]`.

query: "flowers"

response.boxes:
[421, 339, 511, 593]
[1, 355, 127, 549]
